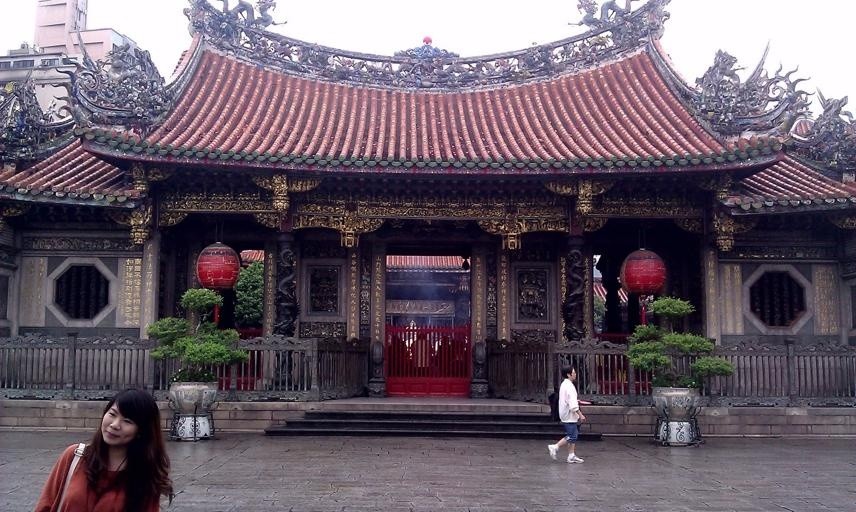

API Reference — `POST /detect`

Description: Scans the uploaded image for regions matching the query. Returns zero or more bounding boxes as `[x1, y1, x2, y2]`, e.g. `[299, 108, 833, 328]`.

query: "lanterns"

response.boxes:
[619, 246, 667, 328]
[196, 241, 240, 325]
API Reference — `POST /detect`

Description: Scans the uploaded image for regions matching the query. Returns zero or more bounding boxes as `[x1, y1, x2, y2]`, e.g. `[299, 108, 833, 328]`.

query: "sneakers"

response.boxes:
[567, 455, 584, 464]
[548, 444, 559, 461]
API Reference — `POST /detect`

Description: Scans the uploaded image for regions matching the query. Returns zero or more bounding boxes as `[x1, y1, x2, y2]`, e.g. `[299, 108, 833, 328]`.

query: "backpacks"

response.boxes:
[548, 392, 561, 421]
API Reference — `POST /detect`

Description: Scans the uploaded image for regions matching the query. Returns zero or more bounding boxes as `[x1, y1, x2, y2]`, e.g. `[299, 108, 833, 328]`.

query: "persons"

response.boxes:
[383, 330, 459, 377]
[548, 364, 588, 463]
[32, 386, 177, 512]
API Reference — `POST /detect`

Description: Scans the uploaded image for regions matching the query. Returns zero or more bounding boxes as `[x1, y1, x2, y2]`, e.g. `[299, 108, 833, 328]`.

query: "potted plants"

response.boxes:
[147, 288, 249, 441]
[625, 295, 735, 447]
